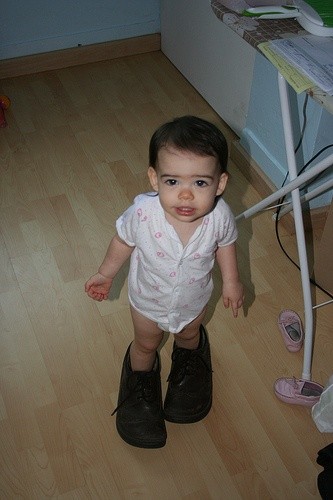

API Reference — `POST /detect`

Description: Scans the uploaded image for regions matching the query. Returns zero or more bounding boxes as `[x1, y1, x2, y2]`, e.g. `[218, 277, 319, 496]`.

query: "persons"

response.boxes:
[83, 115, 246, 452]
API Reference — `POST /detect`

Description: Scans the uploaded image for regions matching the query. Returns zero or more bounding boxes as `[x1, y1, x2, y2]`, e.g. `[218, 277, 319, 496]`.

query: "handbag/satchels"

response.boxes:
[311, 375, 333, 434]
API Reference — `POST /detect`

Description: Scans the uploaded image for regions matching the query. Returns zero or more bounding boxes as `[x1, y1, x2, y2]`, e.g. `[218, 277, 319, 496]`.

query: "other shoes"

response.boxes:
[278, 308, 303, 353]
[273, 377, 325, 407]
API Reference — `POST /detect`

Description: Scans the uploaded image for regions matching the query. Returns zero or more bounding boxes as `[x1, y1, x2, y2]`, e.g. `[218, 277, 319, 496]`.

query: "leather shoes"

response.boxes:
[164, 323, 214, 424]
[116, 340, 168, 449]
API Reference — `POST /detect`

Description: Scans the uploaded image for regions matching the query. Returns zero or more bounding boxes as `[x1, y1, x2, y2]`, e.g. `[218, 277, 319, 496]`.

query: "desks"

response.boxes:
[209, 0, 333, 380]
